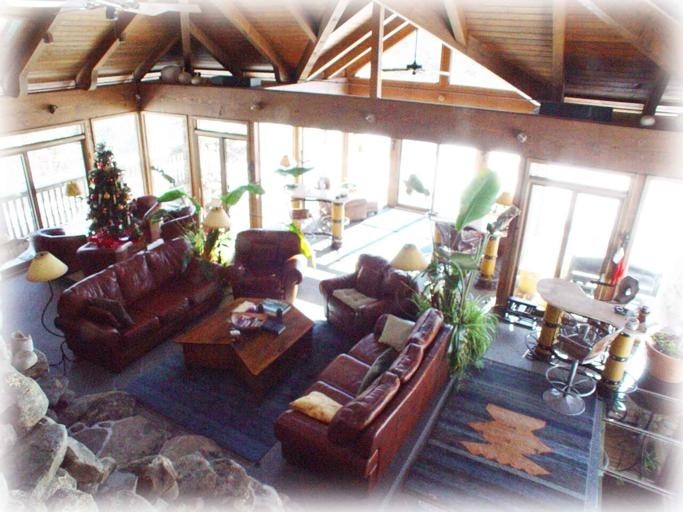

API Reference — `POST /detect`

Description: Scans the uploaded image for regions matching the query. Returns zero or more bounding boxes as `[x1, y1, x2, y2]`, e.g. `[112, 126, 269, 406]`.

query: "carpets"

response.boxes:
[385, 353, 600, 511]
[124, 318, 359, 461]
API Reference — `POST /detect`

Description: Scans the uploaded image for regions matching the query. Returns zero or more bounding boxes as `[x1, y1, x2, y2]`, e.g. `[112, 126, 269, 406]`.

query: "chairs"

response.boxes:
[124, 194, 160, 243]
[542, 322, 625, 417]
[319, 253, 419, 342]
[31, 227, 87, 278]
[74, 239, 133, 277]
[159, 202, 200, 239]
[584, 276, 640, 351]
[227, 228, 304, 302]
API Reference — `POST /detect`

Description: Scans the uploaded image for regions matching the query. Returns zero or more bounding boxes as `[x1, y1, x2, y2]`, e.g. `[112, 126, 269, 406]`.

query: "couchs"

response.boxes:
[49, 234, 225, 373]
[273, 308, 456, 499]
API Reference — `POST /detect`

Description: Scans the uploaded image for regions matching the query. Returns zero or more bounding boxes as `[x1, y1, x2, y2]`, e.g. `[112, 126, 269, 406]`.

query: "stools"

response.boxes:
[368, 202, 377, 215]
[343, 197, 368, 223]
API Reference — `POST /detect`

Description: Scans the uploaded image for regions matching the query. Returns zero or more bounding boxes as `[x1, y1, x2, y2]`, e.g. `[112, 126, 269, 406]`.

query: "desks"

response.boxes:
[289, 186, 352, 250]
[177, 295, 316, 405]
[527, 277, 655, 398]
[425, 212, 510, 292]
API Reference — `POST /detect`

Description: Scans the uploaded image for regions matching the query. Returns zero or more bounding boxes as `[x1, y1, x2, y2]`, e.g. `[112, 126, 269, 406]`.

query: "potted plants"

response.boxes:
[403, 166, 504, 395]
[645, 326, 682, 389]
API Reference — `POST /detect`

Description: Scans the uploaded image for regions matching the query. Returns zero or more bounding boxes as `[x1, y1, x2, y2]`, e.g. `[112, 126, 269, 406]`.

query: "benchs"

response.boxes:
[565, 258, 655, 300]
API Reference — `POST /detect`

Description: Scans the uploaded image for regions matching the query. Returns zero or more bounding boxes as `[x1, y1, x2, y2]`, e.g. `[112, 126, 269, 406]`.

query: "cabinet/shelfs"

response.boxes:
[604, 370, 683, 503]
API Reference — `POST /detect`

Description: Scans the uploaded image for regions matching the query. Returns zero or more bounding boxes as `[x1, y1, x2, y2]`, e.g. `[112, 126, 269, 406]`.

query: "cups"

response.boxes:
[625, 316, 639, 331]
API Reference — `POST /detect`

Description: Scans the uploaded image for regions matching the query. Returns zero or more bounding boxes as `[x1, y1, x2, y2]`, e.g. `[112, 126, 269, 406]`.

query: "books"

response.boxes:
[226, 294, 292, 341]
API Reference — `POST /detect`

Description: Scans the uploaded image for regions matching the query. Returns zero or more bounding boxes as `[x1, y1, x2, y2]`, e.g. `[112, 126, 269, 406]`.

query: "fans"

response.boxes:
[26, 3, 202, 26]
[382, 28, 455, 80]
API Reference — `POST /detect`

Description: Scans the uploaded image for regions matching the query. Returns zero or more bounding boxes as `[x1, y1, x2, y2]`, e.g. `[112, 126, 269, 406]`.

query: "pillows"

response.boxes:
[354, 265, 381, 296]
[357, 345, 393, 397]
[86, 296, 136, 329]
[86, 306, 118, 329]
[378, 314, 416, 348]
[287, 392, 343, 425]
[333, 287, 377, 311]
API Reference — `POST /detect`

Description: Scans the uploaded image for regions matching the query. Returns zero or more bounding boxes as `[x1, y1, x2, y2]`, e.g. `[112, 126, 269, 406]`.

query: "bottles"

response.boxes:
[636, 305, 650, 325]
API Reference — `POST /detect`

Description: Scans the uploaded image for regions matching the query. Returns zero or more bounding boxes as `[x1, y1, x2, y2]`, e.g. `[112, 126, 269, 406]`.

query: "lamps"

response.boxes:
[66, 181, 85, 221]
[495, 189, 512, 218]
[203, 206, 232, 269]
[25, 251, 75, 374]
[392, 243, 426, 284]
